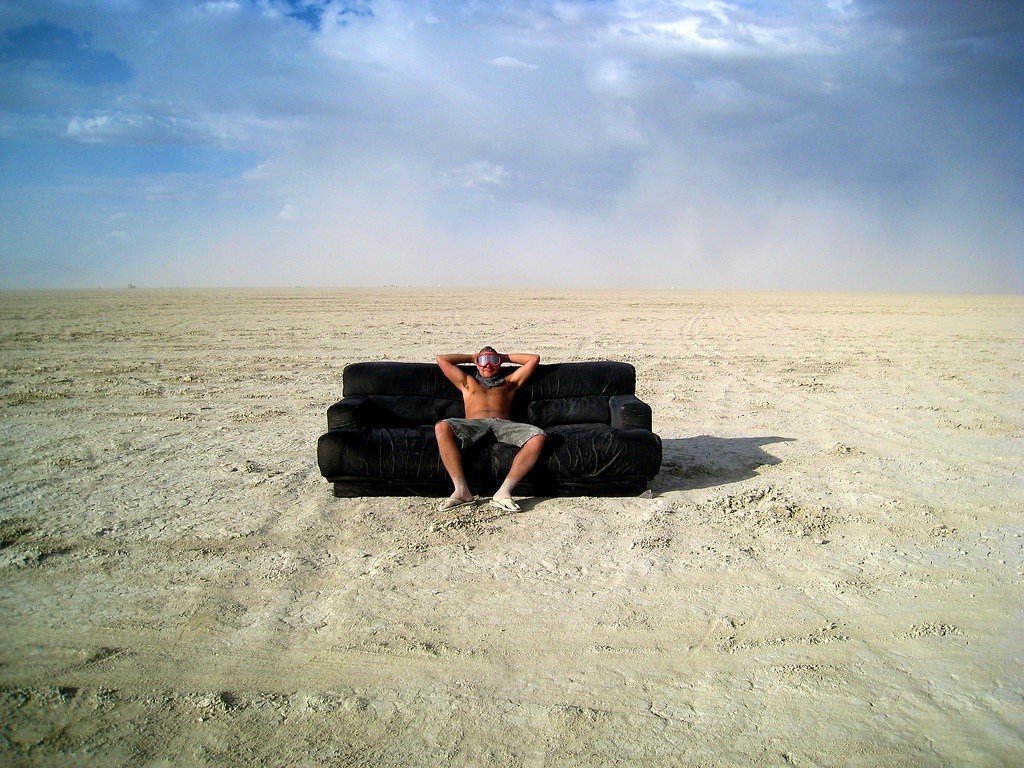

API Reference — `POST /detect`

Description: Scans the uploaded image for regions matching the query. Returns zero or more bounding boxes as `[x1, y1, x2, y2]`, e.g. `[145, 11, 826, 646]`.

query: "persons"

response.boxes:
[434, 346, 547, 512]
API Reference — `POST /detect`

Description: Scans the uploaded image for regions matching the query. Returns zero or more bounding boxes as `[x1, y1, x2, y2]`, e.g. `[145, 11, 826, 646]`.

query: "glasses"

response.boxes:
[473, 352, 500, 367]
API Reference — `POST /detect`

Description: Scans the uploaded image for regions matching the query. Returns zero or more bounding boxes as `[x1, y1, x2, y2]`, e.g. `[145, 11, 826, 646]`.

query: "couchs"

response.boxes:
[317, 361, 662, 499]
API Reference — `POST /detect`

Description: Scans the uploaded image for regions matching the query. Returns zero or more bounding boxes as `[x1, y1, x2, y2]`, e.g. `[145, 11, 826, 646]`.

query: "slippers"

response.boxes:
[437, 498, 476, 512]
[489, 498, 521, 512]
